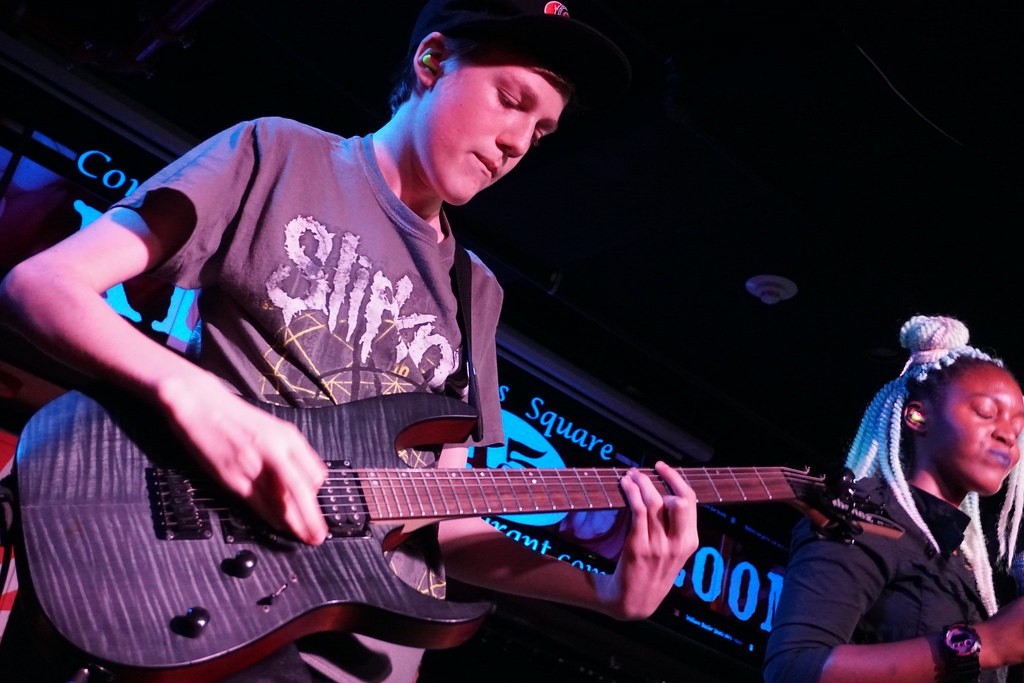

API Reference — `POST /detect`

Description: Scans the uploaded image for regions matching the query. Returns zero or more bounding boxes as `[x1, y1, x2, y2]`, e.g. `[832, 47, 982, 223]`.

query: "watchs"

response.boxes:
[939, 622, 981, 683]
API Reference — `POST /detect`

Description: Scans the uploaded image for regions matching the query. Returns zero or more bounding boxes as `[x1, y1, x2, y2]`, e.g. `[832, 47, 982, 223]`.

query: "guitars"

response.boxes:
[11, 387, 908, 681]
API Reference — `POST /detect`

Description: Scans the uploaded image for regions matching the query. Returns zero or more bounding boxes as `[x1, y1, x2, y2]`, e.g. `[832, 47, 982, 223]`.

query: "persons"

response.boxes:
[762, 316, 1024, 683]
[0, 0, 700, 682]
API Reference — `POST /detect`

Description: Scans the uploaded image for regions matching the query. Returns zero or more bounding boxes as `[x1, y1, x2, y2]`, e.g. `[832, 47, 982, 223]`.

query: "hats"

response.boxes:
[409, 0, 631, 132]
